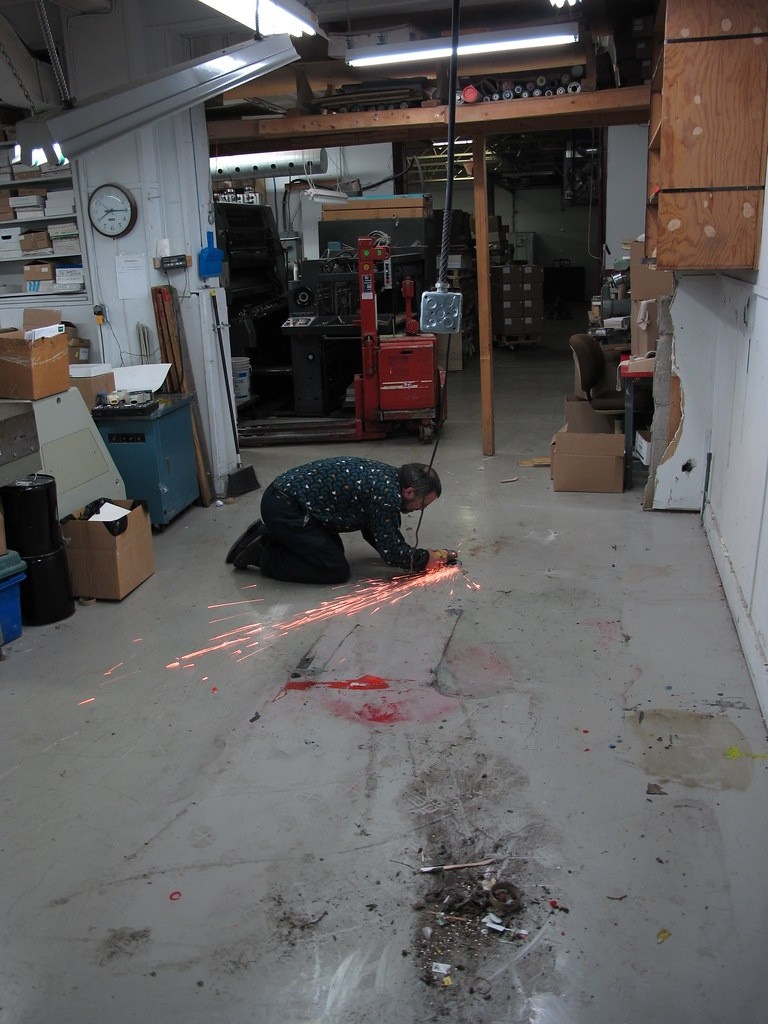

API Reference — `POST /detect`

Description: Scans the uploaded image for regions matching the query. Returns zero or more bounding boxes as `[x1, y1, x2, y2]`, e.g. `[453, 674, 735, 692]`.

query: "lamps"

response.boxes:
[198, 0, 329, 41]
[345, 22, 584, 67]
[10, 32, 302, 166]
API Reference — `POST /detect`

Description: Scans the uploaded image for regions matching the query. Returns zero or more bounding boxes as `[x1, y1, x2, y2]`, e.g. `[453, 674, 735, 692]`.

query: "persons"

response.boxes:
[226, 456, 446, 585]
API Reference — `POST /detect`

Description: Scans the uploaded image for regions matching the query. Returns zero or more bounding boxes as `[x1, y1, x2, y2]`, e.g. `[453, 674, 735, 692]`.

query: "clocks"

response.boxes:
[88, 183, 138, 238]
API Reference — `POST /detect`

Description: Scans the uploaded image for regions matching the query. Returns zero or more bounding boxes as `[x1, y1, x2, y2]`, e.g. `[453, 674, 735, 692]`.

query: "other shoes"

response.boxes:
[233, 534, 264, 569]
[225, 517, 266, 563]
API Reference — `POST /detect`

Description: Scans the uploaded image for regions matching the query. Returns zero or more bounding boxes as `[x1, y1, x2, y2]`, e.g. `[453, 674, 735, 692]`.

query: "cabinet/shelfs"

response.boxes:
[644, 0, 768, 270]
[0, 141, 93, 308]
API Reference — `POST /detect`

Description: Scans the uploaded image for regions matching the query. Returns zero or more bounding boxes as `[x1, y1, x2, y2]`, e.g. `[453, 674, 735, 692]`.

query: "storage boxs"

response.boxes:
[328, 24, 430, 59]
[18, 229, 52, 251]
[550, 239, 675, 493]
[0, 307, 70, 400]
[24, 260, 54, 281]
[488, 213, 515, 255]
[62, 321, 78, 339]
[61, 499, 156, 601]
[491, 264, 547, 336]
[67, 339, 91, 364]
[70, 372, 115, 414]
[437, 331, 464, 372]
